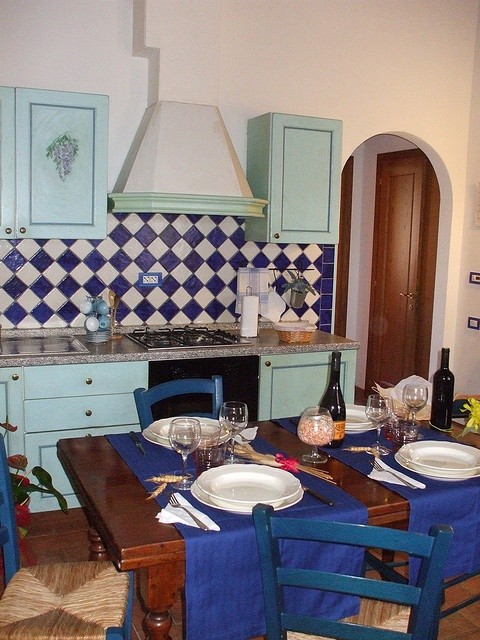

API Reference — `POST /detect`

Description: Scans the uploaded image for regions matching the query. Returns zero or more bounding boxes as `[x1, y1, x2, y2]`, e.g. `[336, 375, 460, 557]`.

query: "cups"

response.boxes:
[85, 317, 99, 332]
[94, 298, 109, 316]
[385, 407, 409, 443]
[400, 420, 420, 444]
[193, 424, 221, 472]
[98, 316, 112, 332]
[80, 299, 93, 315]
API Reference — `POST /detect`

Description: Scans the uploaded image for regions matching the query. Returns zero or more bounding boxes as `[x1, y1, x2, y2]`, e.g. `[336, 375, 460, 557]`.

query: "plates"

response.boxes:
[395, 451, 480, 482]
[196, 463, 301, 512]
[398, 441, 480, 478]
[190, 480, 305, 515]
[149, 416, 230, 440]
[345, 404, 384, 434]
[142, 416, 233, 450]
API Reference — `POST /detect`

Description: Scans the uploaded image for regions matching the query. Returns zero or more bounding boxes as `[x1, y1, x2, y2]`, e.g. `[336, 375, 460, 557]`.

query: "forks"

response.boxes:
[367, 456, 418, 491]
[164, 489, 212, 532]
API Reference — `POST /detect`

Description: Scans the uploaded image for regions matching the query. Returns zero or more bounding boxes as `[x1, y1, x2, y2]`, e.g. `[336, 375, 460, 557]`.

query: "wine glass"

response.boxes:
[403, 383, 428, 441]
[365, 395, 394, 456]
[168, 417, 202, 491]
[219, 401, 249, 465]
[297, 407, 335, 464]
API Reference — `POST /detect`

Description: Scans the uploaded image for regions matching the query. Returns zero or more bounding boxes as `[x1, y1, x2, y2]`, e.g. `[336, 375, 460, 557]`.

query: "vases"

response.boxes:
[14, 495, 34, 527]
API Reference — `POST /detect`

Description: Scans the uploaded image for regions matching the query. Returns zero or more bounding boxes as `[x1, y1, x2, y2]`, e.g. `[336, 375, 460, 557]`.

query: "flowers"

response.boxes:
[0, 422, 69, 516]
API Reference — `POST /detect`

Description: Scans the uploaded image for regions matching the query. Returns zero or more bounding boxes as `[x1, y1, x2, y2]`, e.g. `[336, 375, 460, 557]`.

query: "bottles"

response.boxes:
[318, 352, 345, 447]
[430, 348, 455, 430]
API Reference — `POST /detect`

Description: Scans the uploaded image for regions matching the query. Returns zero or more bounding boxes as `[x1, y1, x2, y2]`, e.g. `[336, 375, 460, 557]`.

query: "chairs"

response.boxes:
[133, 373, 226, 433]
[0, 433, 20, 587]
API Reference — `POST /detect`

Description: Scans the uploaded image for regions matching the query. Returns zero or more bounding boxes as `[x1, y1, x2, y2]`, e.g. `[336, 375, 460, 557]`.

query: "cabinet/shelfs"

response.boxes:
[244, 111, 344, 246]
[255, 349, 362, 421]
[0, 86, 111, 242]
[25, 361, 152, 513]
[1, 368, 25, 471]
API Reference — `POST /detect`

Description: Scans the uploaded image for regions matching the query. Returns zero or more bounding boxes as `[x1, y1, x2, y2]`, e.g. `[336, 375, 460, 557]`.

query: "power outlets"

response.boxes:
[466, 315, 480, 330]
[138, 272, 163, 288]
[468, 271, 480, 286]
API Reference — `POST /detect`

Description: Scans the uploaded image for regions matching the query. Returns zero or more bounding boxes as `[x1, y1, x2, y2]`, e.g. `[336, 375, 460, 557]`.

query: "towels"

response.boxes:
[366, 456, 426, 490]
[232, 425, 259, 446]
[155, 491, 221, 533]
